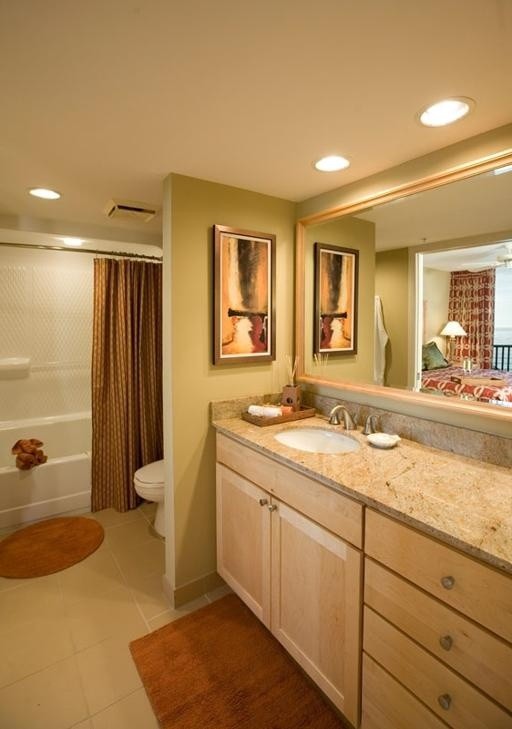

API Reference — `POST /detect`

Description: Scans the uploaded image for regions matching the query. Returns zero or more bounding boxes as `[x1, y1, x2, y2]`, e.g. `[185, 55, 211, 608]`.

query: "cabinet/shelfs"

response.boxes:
[215, 430, 365, 729]
[362, 506, 512, 729]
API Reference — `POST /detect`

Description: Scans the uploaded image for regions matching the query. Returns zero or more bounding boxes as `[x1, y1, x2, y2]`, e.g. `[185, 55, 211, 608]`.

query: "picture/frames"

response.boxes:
[314, 241, 359, 358]
[213, 224, 276, 367]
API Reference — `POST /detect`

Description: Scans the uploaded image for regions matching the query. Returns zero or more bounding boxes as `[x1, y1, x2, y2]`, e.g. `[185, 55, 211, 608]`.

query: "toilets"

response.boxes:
[133, 458, 170, 539]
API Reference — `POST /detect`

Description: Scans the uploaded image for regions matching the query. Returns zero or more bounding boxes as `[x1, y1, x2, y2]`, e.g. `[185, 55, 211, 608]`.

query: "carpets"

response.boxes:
[0, 517, 104, 579]
[128, 594, 354, 729]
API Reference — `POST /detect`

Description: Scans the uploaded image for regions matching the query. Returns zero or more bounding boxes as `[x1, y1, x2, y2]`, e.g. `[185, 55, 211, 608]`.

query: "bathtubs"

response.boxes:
[0, 409, 91, 529]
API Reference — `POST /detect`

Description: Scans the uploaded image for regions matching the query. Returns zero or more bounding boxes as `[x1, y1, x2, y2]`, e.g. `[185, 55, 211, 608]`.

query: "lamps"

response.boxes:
[440, 321, 467, 363]
[498, 245, 512, 268]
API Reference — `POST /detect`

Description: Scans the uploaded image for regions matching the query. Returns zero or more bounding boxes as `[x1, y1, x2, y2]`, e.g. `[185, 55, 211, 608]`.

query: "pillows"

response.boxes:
[422, 341, 448, 371]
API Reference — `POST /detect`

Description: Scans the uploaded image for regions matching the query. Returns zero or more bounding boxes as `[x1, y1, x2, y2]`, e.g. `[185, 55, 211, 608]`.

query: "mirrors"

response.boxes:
[294, 147, 512, 424]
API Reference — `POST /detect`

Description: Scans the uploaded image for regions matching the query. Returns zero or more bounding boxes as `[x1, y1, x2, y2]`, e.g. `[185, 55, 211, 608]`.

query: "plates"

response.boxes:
[366, 432, 401, 449]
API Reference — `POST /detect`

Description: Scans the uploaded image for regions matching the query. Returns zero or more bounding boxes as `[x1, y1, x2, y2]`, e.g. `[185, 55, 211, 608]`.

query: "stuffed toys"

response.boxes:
[12, 438, 48, 471]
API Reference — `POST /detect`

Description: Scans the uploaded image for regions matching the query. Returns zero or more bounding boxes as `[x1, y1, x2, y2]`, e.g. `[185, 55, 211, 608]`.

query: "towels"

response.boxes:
[248, 406, 282, 416]
[374, 295, 389, 386]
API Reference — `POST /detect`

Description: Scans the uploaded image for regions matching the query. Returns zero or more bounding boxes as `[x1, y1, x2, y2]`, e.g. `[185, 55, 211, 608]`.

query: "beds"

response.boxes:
[421, 366, 512, 407]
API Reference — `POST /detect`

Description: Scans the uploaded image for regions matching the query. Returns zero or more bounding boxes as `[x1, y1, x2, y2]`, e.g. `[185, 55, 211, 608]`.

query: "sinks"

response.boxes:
[272, 422, 365, 455]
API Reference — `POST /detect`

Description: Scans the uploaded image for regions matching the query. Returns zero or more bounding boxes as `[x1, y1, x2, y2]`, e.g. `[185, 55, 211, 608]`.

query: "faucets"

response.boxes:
[329, 405, 362, 430]
